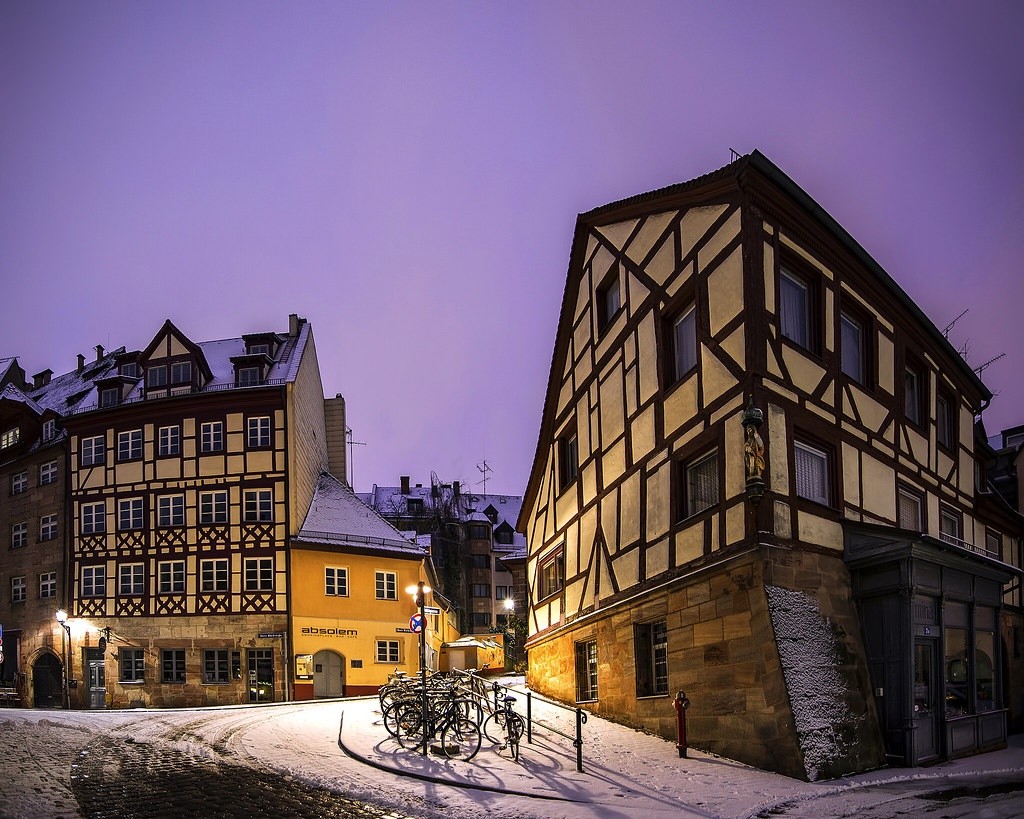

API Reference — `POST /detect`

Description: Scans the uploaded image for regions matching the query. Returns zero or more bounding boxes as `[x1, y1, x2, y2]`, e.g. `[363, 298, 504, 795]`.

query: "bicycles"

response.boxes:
[376, 663, 526, 762]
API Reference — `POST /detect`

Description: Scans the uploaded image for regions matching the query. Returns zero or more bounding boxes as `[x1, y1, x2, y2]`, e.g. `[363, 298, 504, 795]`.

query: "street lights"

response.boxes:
[504, 596, 516, 631]
[405, 585, 433, 756]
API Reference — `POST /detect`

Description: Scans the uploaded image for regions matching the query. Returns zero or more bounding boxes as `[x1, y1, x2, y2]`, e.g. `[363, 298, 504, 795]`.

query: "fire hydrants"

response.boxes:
[671, 689, 691, 757]
